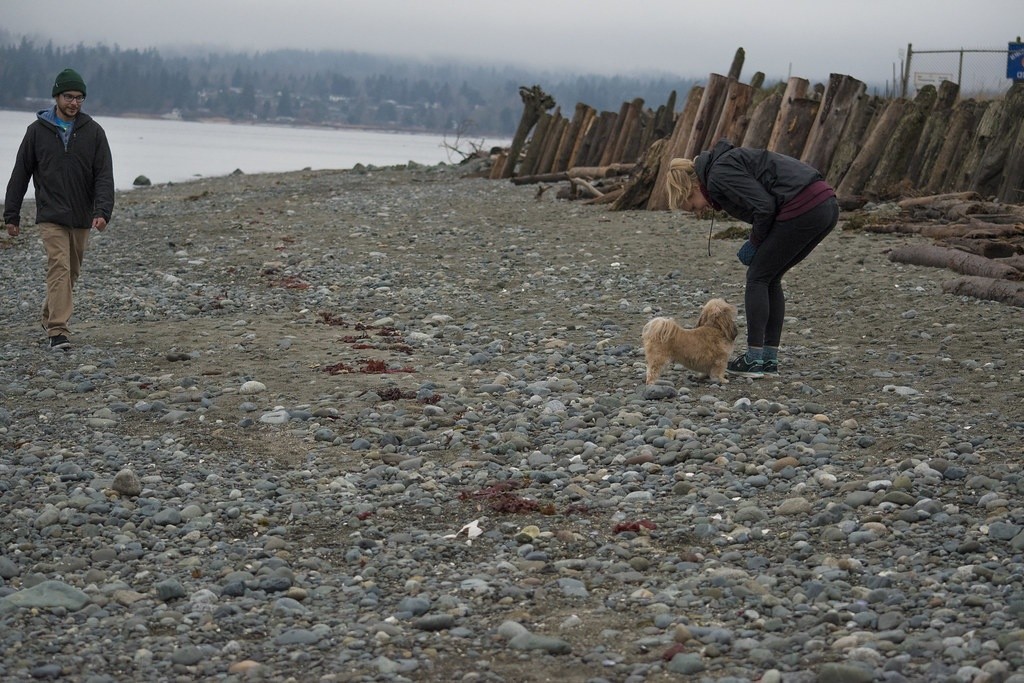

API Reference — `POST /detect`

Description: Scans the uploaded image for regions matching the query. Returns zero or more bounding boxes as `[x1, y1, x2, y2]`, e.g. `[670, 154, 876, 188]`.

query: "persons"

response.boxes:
[667, 138, 840, 378]
[3, 66, 115, 348]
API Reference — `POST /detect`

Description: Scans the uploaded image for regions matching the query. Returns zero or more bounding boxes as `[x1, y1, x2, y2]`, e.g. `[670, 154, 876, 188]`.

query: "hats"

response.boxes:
[52, 68, 88, 97]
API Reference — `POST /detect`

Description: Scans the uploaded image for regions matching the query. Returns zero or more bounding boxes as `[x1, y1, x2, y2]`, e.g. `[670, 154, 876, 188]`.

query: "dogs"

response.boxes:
[641, 297, 738, 386]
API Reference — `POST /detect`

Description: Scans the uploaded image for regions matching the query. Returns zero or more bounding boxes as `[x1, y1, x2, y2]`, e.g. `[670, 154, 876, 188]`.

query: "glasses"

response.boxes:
[62, 93, 86, 103]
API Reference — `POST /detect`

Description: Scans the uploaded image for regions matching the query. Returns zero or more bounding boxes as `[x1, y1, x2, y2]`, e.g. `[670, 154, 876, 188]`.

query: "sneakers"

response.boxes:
[48, 335, 72, 350]
[763, 359, 780, 377]
[41, 323, 48, 333]
[726, 356, 765, 378]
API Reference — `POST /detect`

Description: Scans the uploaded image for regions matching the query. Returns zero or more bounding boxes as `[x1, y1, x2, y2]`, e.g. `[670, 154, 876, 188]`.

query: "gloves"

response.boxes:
[737, 241, 757, 265]
